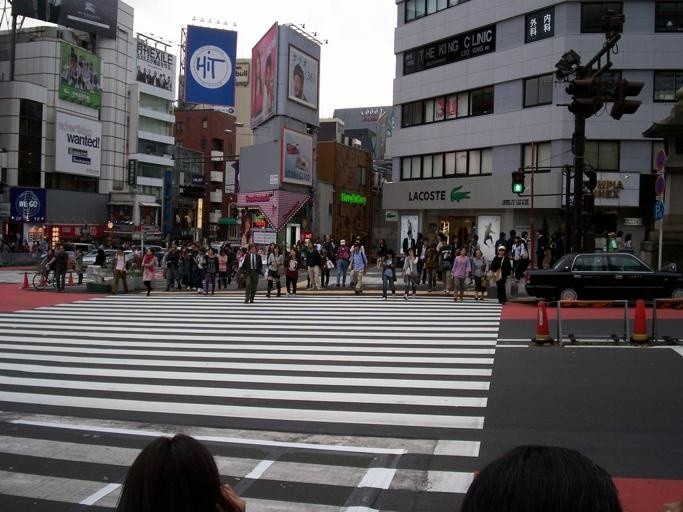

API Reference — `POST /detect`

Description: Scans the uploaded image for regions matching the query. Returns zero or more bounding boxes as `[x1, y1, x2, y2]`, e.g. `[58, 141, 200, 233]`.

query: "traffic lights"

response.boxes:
[178, 184, 207, 200]
[511, 172, 524, 194]
[611, 79, 645, 120]
[564, 79, 602, 119]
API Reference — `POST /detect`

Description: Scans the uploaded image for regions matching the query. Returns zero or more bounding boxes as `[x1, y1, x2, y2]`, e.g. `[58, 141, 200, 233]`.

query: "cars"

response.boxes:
[81, 244, 166, 270]
[525, 252, 682, 309]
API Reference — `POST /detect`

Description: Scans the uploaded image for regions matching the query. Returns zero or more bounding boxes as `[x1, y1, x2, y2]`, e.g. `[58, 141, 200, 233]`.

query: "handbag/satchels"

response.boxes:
[326, 260, 334, 269]
[446, 274, 453, 291]
[486, 268, 502, 282]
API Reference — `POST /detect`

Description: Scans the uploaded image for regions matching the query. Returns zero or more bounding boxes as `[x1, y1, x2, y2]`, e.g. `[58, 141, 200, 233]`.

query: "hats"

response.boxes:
[340, 240, 346, 245]
[497, 246, 506, 251]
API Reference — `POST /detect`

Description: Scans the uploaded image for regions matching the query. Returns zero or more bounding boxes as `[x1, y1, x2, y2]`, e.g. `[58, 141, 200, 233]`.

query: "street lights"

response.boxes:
[554, 10, 625, 257]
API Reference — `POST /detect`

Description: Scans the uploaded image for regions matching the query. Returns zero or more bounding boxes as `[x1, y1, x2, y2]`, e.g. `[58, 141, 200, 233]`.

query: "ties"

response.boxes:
[251, 255, 253, 269]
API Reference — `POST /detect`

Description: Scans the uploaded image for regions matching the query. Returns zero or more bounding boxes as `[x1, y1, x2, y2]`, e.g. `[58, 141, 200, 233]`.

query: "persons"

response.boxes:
[41, 246, 68, 292]
[472, 249, 488, 300]
[403, 230, 633, 300]
[94, 249, 106, 265]
[490, 246, 511, 302]
[452, 246, 471, 301]
[290, 60, 308, 104]
[134, 66, 175, 91]
[239, 244, 262, 302]
[162, 236, 386, 297]
[92, 243, 138, 255]
[141, 249, 155, 296]
[114, 432, 245, 512]
[381, 249, 396, 300]
[252, 52, 264, 116]
[60, 51, 99, 97]
[111, 247, 128, 293]
[3, 240, 40, 253]
[483, 223, 497, 248]
[407, 219, 415, 239]
[452, 443, 623, 511]
[261, 55, 276, 113]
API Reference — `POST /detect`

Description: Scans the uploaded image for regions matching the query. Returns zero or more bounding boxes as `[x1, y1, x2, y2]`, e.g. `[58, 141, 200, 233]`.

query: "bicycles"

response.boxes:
[32, 263, 58, 291]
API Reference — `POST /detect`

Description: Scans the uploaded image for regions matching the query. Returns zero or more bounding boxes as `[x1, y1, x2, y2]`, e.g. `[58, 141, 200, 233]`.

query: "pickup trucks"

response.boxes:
[40, 242, 95, 271]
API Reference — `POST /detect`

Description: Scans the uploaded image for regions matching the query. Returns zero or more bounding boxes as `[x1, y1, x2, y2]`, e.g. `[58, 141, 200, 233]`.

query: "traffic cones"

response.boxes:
[20, 272, 31, 288]
[37, 275, 47, 286]
[65, 272, 75, 285]
[531, 298, 553, 344]
[631, 299, 650, 342]
